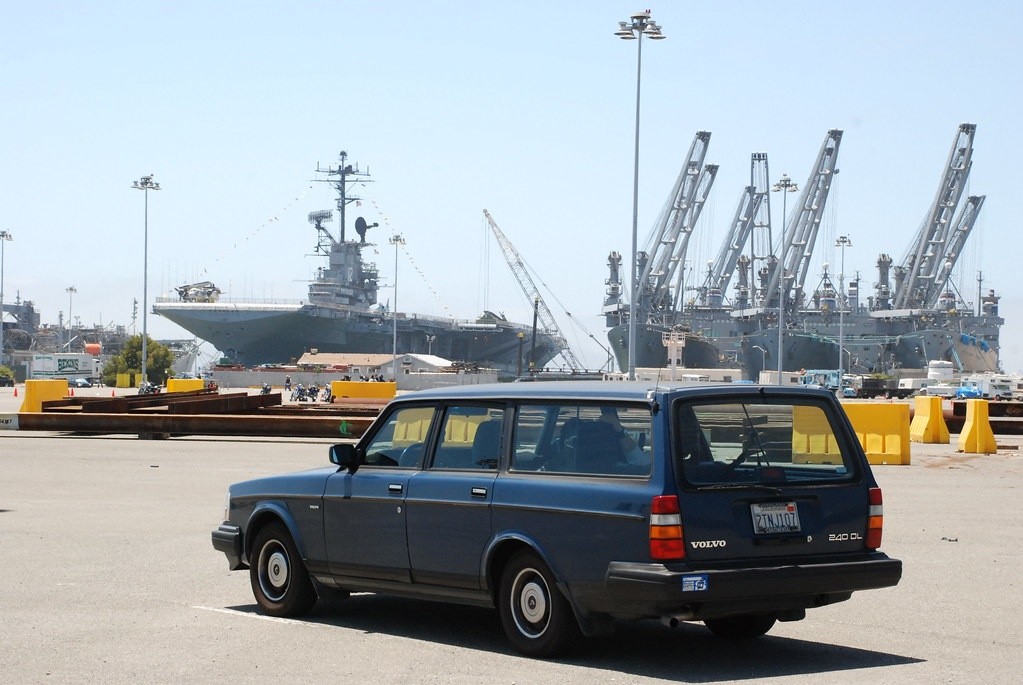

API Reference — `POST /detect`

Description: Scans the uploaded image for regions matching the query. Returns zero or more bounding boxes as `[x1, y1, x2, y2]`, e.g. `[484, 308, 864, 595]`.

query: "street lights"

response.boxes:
[132, 173, 163, 393]
[821, 262, 830, 333]
[0, 227, 15, 365]
[772, 171, 801, 387]
[707, 259, 715, 337]
[66, 285, 77, 353]
[389, 231, 407, 382]
[614, 6, 667, 380]
[944, 259, 954, 328]
[835, 234, 853, 398]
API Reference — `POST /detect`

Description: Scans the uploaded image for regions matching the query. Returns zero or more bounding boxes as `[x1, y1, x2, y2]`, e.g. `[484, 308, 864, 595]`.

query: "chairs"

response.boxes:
[471, 420, 506, 467]
[543, 418, 584, 460]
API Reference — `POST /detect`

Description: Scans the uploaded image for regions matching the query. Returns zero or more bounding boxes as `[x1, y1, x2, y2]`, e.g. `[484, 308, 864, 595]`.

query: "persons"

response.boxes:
[359, 374, 385, 382]
[597, 413, 651, 466]
[285, 375, 292, 391]
[340, 375, 351, 381]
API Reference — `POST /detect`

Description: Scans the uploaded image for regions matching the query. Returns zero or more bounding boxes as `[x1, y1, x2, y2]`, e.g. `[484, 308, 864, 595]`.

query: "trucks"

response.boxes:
[26, 354, 103, 383]
[845, 372, 1023, 401]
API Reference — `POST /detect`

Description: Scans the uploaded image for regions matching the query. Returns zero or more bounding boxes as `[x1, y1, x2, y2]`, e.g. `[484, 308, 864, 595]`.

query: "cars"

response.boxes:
[165, 369, 213, 379]
[72, 377, 92, 388]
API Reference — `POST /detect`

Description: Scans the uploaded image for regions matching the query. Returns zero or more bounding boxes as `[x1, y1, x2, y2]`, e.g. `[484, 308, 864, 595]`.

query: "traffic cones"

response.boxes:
[14, 388, 17, 397]
[112, 391, 114, 396]
[71, 388, 74, 395]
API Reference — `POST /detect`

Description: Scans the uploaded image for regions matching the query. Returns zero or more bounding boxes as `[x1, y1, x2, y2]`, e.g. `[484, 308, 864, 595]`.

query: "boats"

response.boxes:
[162, 157, 566, 374]
[605, 123, 1002, 368]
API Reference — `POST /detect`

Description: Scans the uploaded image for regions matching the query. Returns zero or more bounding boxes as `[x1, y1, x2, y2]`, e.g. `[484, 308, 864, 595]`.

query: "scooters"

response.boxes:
[290, 383, 331, 402]
[138, 381, 162, 394]
[260, 381, 273, 394]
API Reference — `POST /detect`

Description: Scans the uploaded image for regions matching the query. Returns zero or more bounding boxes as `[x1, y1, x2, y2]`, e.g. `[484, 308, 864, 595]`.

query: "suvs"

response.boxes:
[210, 381, 903, 658]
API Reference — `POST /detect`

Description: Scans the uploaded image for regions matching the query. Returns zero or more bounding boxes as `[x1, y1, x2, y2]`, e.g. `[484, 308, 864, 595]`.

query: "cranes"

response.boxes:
[482, 213, 607, 373]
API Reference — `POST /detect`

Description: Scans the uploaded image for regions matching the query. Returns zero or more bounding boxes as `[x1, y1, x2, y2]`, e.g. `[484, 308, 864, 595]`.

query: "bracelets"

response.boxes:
[615, 426, 627, 440]
